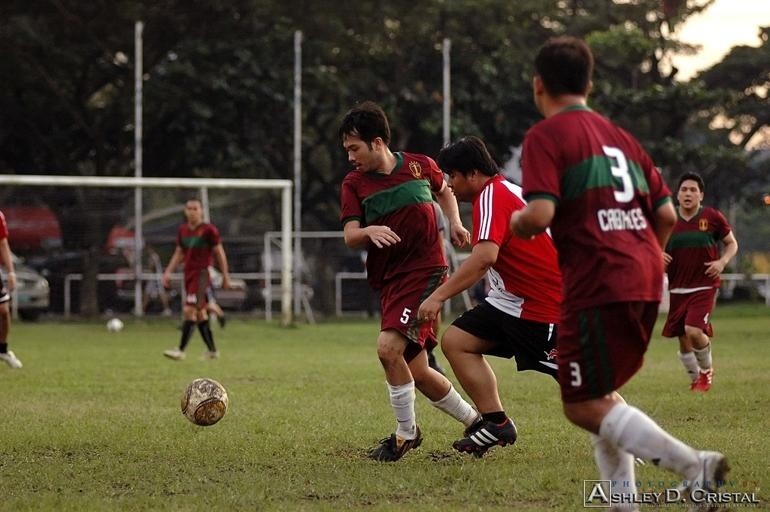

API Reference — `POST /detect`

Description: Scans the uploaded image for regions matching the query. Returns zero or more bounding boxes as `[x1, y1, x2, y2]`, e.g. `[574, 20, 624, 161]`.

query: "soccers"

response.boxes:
[108, 318, 123, 332]
[181, 378, 228, 426]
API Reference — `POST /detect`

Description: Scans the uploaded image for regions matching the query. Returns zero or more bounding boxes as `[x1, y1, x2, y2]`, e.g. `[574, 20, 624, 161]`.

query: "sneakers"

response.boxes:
[673, 449, 730, 512]
[687, 370, 698, 391]
[694, 367, 714, 393]
[0, 349, 23, 369]
[370, 425, 424, 464]
[452, 418, 519, 454]
[197, 350, 220, 361]
[130, 307, 146, 317]
[160, 308, 173, 318]
[162, 346, 186, 361]
[217, 314, 226, 328]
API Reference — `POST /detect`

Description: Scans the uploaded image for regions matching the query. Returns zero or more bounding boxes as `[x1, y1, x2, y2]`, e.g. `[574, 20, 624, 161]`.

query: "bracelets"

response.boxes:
[7, 271, 15, 276]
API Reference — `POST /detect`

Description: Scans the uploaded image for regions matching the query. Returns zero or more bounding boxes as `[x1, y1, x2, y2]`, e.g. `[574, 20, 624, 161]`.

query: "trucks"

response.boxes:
[0, 195, 63, 301]
[719, 273, 769, 304]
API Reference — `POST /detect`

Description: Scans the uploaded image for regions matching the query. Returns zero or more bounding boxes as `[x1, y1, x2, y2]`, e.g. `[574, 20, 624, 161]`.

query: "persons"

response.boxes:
[0, 210, 23, 370]
[162, 197, 231, 361]
[122, 245, 172, 318]
[339, 34, 739, 512]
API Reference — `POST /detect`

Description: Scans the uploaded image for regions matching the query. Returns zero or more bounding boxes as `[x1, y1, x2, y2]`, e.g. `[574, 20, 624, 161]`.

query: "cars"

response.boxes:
[1, 249, 51, 323]
[113, 244, 364, 311]
